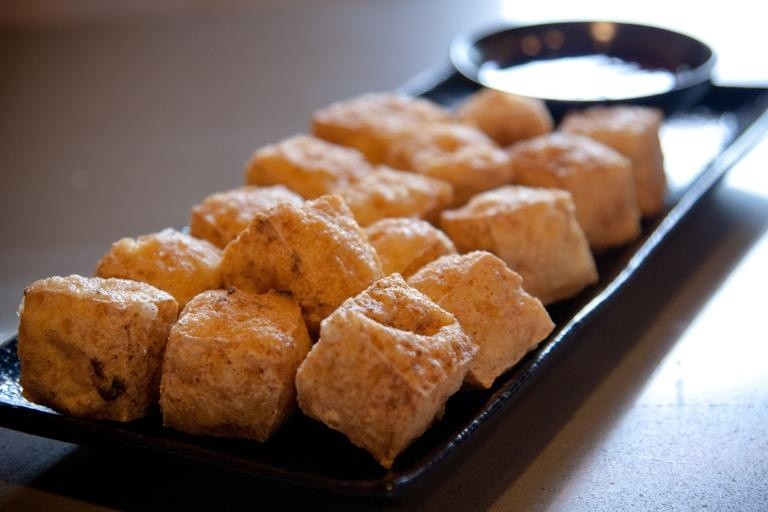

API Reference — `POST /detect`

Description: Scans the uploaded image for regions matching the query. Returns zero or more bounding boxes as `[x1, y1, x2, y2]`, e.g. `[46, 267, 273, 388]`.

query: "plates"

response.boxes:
[2, 46, 765, 487]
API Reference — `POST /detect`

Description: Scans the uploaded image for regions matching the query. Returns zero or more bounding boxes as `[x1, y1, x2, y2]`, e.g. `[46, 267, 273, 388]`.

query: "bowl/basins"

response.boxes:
[448, 20, 718, 127]
